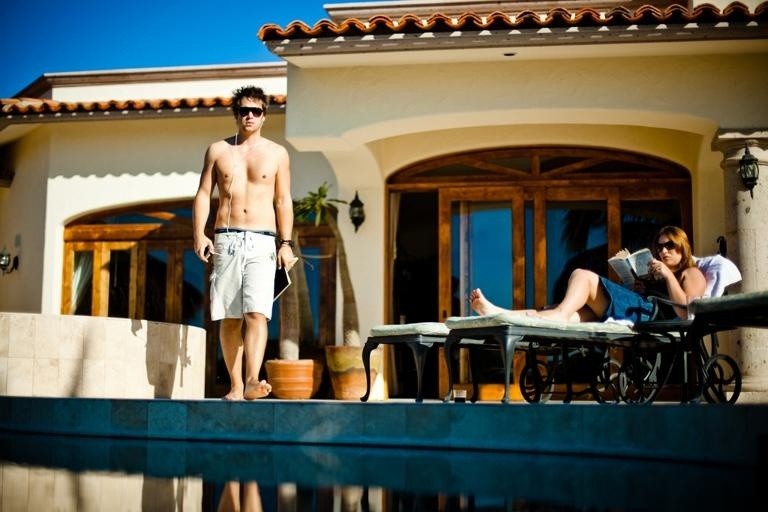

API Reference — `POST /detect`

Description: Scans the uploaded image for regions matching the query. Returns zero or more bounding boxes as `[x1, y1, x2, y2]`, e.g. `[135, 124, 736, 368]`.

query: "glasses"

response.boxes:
[656, 241, 674, 253]
[237, 107, 263, 116]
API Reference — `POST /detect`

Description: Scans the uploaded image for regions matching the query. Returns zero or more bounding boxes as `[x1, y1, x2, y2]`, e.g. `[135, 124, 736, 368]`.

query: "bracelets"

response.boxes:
[280, 238, 294, 248]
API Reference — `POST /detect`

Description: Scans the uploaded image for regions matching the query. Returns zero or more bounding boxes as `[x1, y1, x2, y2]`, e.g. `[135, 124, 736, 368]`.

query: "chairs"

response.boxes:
[441, 253, 743, 405]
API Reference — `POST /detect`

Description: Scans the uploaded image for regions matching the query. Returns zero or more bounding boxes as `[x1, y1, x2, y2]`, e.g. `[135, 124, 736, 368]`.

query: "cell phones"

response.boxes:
[204, 245, 210, 259]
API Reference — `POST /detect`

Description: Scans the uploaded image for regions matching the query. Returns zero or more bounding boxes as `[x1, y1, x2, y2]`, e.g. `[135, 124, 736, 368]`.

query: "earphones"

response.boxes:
[237, 115, 239, 120]
[228, 233, 230, 239]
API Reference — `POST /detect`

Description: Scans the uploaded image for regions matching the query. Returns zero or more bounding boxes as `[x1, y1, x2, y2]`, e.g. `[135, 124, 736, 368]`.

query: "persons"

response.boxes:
[192, 86, 295, 402]
[468, 226, 707, 325]
[216, 482, 263, 512]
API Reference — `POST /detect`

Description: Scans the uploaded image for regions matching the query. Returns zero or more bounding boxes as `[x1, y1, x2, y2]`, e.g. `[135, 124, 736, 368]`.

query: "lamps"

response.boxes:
[737, 141, 759, 199]
[0, 243, 19, 277]
[348, 190, 364, 233]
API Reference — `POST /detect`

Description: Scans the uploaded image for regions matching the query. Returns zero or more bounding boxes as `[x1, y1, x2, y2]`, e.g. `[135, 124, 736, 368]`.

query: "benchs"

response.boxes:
[358, 322, 553, 404]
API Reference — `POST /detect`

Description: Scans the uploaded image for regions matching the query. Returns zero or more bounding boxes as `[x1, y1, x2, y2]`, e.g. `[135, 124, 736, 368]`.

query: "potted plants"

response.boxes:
[265, 268, 324, 400]
[292, 179, 383, 401]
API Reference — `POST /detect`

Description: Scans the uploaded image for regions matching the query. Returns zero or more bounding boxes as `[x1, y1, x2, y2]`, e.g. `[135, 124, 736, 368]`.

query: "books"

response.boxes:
[608, 247, 655, 288]
[273, 255, 299, 302]
